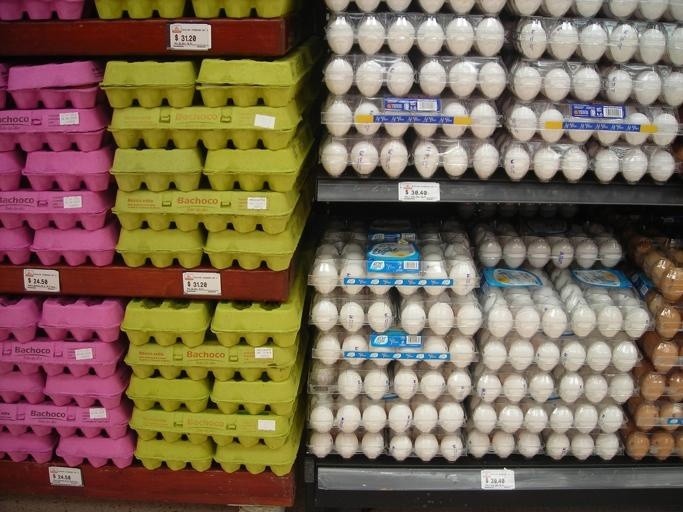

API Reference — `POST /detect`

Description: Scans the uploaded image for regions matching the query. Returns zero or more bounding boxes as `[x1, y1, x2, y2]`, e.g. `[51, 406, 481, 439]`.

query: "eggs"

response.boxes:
[323, 1, 683, 183]
[310, 203, 683, 462]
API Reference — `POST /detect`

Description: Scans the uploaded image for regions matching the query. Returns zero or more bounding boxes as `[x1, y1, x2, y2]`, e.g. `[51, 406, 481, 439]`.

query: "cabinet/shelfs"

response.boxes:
[0, 16, 683, 510]
